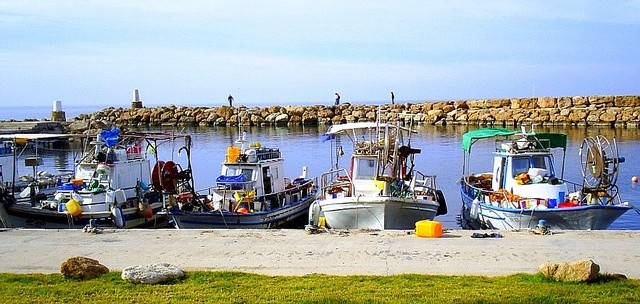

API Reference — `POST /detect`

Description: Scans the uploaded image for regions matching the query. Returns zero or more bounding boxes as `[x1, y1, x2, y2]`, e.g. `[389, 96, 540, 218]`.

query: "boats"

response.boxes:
[0, 127, 193, 228]
[318, 104, 440, 230]
[459, 124, 633, 230]
[157, 118, 319, 229]
